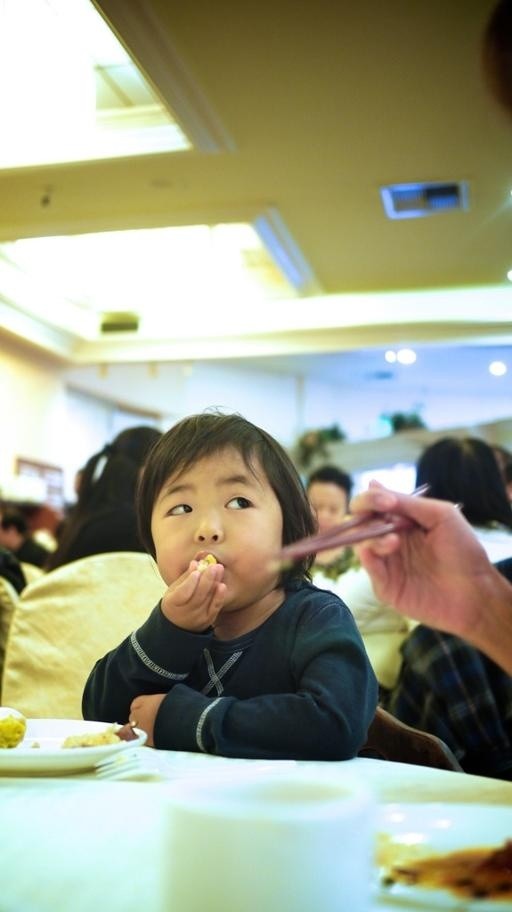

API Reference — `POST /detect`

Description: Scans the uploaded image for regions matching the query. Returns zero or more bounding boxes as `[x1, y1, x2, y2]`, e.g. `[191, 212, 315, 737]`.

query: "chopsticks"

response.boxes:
[270, 482, 465, 558]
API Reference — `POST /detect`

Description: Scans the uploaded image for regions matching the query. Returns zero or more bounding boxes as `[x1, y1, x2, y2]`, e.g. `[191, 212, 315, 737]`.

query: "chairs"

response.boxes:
[1, 550, 170, 720]
[370, 704, 465, 772]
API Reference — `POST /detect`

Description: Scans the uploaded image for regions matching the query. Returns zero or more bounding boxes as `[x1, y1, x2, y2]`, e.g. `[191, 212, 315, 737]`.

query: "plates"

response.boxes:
[380, 845, 512, 912]
[0, 716, 147, 776]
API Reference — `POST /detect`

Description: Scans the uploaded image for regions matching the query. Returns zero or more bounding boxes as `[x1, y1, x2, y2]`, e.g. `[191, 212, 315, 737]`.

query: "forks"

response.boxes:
[94, 746, 149, 781]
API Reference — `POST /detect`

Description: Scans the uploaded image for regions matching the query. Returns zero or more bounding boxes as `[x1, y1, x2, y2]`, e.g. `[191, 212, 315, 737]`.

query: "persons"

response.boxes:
[358, 557, 511, 782]
[43, 426, 164, 572]
[0, 511, 51, 595]
[81, 414, 379, 760]
[307, 464, 361, 584]
[329, 434, 512, 690]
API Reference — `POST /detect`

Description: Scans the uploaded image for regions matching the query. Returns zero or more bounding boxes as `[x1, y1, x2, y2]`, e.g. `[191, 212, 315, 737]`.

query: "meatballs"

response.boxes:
[0, 706, 26, 748]
